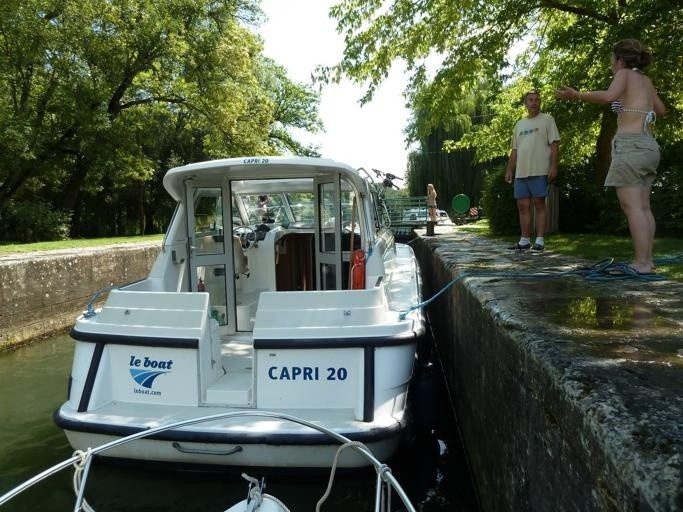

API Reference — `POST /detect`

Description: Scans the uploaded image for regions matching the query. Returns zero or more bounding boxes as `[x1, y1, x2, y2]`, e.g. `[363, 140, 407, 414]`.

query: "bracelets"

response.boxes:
[578, 92, 583, 101]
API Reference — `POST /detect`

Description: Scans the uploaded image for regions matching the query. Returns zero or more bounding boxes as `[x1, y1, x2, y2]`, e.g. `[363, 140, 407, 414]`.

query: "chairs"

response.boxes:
[202, 236, 249, 306]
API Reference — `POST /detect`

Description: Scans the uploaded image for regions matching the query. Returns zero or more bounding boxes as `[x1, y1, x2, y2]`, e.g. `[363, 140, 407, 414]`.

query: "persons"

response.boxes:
[556, 39, 666, 277]
[427, 183, 437, 221]
[505, 91, 561, 252]
[257, 194, 269, 219]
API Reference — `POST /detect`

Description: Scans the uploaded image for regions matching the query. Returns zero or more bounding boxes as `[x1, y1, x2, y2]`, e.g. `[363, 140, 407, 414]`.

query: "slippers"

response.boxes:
[606, 263, 657, 276]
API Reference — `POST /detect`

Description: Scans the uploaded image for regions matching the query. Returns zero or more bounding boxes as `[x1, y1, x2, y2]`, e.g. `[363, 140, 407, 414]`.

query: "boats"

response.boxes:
[51, 154, 425, 468]
[399, 207, 455, 226]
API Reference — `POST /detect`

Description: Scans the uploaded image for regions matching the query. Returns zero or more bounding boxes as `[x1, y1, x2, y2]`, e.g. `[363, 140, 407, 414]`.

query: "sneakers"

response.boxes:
[505, 243, 545, 254]
